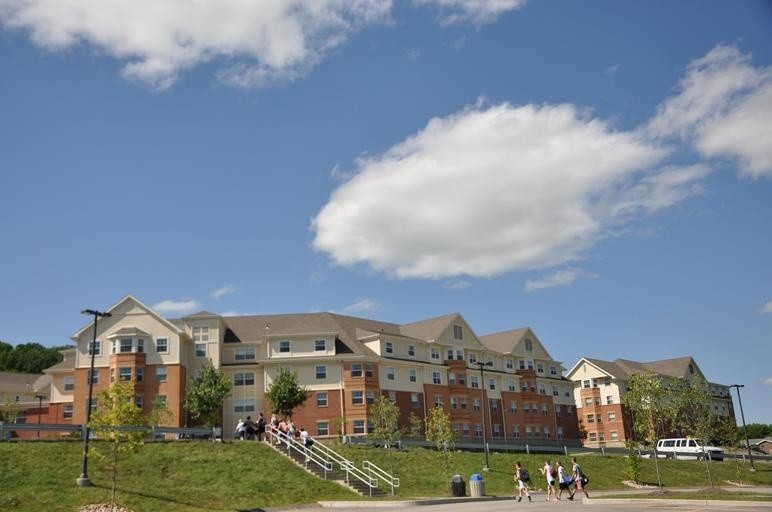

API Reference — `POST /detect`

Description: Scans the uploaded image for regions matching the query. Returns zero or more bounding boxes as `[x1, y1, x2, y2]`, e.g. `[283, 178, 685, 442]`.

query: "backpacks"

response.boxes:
[516, 468, 530, 481]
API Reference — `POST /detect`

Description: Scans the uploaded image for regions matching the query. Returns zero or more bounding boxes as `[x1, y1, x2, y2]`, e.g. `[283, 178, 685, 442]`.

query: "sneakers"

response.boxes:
[276, 440, 289, 449]
[518, 496, 531, 502]
[546, 495, 573, 501]
[304, 458, 310, 463]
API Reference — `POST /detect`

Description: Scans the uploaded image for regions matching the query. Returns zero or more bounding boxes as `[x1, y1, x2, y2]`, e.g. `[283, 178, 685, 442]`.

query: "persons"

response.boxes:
[567, 457, 591, 499]
[538, 459, 559, 501]
[513, 461, 533, 502]
[554, 460, 572, 500]
[233, 407, 314, 463]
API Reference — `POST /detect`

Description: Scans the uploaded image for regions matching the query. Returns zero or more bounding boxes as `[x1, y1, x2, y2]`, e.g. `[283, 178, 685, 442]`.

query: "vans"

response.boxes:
[655, 437, 725, 461]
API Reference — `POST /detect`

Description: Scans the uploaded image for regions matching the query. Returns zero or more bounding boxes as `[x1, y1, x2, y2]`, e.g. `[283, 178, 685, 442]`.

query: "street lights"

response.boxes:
[730, 384, 755, 469]
[81, 308, 112, 478]
[472, 360, 493, 470]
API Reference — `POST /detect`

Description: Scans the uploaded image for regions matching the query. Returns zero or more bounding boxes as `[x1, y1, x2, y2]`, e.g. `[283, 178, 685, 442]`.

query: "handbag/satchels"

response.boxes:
[306, 436, 314, 446]
[295, 431, 300, 437]
[564, 475, 575, 487]
[549, 468, 558, 478]
[581, 473, 589, 486]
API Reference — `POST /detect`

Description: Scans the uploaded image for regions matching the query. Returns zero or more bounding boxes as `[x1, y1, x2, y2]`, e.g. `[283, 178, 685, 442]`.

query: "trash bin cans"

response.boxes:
[451, 474, 484, 497]
